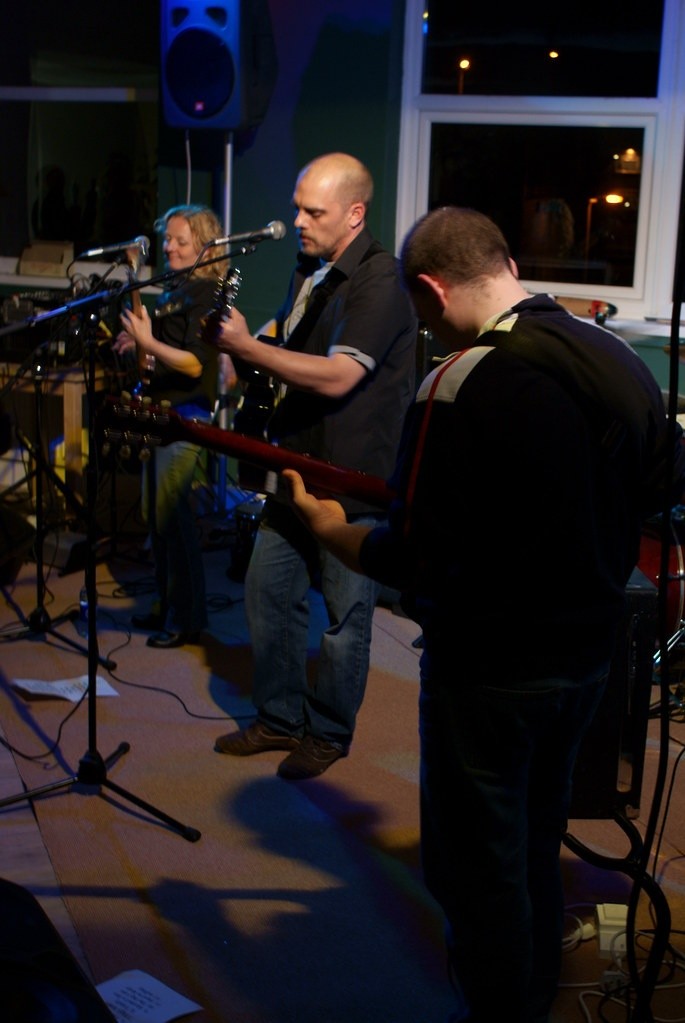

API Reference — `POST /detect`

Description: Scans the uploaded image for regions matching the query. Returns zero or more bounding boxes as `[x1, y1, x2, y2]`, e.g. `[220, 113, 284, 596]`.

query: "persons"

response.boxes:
[112, 205, 231, 648]
[273, 206, 666, 1023]
[213, 151, 415, 781]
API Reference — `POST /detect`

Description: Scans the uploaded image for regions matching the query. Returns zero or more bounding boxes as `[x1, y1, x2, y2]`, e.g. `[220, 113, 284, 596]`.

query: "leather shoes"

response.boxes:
[131, 611, 165, 630]
[147, 626, 202, 647]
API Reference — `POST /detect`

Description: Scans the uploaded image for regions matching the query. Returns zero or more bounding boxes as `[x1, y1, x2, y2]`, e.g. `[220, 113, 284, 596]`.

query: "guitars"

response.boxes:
[90, 390, 402, 514]
[212, 262, 286, 494]
[122, 244, 158, 388]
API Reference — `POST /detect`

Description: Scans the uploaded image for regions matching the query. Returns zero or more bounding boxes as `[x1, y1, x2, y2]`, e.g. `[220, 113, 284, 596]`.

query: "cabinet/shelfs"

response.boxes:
[0, 86, 161, 299]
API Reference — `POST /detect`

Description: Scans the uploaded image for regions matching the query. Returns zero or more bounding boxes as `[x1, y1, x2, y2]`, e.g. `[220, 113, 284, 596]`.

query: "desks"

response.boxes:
[0, 362, 105, 522]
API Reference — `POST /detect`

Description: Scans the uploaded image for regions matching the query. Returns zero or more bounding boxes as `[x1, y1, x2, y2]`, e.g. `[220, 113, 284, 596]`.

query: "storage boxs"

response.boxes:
[18, 240, 74, 276]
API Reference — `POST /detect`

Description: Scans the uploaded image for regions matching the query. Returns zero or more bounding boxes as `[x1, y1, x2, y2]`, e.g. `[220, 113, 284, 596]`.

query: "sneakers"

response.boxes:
[278, 738, 340, 779]
[217, 723, 298, 755]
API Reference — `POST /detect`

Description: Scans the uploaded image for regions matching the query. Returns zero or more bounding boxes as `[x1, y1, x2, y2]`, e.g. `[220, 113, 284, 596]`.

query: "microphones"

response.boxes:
[80, 235, 150, 257]
[209, 221, 286, 247]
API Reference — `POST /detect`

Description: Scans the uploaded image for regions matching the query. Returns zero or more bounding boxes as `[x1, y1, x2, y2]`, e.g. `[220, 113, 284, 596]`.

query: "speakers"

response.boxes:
[161, 0, 248, 130]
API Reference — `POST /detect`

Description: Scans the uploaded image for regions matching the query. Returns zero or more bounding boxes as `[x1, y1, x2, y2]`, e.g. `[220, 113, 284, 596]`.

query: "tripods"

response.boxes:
[0, 254, 259, 842]
